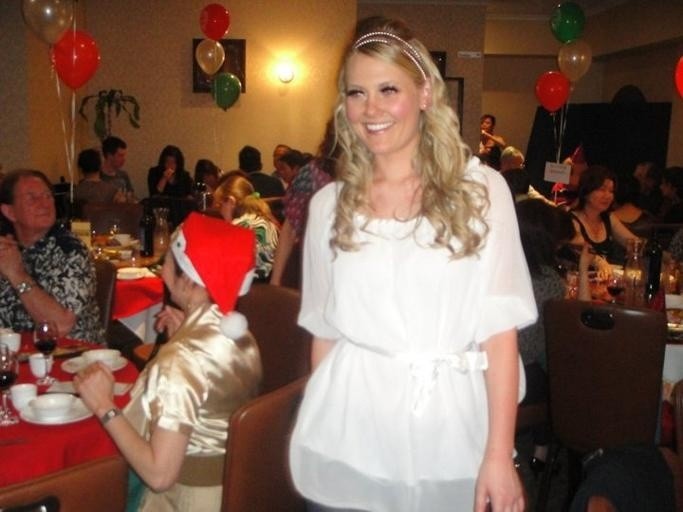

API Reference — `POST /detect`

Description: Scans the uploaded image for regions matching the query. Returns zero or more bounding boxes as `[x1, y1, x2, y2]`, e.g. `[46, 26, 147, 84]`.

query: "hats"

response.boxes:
[167, 208, 260, 342]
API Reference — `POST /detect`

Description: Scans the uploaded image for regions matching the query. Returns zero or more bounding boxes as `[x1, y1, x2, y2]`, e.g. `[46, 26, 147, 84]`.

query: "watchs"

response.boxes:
[99, 408, 121, 427]
[13, 279, 34, 297]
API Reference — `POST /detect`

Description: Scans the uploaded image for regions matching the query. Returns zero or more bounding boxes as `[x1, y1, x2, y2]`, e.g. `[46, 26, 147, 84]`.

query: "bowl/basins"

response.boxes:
[30, 393, 76, 420]
[84, 347, 121, 368]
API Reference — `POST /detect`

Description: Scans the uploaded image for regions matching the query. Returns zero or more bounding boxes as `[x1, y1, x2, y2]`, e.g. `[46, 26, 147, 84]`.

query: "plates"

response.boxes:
[60, 357, 128, 377]
[21, 398, 91, 425]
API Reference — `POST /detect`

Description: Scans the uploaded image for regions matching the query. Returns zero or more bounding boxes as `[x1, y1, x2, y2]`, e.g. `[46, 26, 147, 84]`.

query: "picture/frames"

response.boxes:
[440, 76, 465, 136]
[425, 50, 446, 76]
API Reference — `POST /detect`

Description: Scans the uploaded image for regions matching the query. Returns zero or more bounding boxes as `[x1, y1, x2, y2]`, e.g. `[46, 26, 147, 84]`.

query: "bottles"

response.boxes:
[153, 207, 170, 250]
[565, 237, 682, 329]
[139, 201, 153, 258]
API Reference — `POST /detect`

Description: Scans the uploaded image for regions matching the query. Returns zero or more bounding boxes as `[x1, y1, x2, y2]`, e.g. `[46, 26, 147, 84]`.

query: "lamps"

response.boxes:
[273, 57, 295, 85]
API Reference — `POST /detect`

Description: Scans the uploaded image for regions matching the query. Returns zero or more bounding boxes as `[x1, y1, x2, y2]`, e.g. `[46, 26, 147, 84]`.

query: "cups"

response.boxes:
[0, 332, 22, 353]
[30, 354, 52, 377]
[9, 383, 38, 411]
[88, 224, 145, 282]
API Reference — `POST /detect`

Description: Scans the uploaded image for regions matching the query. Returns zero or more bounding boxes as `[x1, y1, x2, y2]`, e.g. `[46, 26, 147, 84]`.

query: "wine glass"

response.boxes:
[33, 318, 59, 383]
[0, 342, 19, 426]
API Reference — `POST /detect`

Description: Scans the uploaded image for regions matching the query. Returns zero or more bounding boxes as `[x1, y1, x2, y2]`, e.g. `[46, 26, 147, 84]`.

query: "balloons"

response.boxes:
[672, 53, 683, 98]
[200, 4, 230, 40]
[557, 43, 593, 83]
[549, 3, 585, 44]
[21, 1, 73, 45]
[196, 39, 226, 76]
[536, 70, 569, 115]
[211, 74, 240, 113]
[48, 29, 100, 91]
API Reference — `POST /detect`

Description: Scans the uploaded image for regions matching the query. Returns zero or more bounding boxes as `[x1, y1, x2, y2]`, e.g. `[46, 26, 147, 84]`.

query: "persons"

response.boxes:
[288, 15, 541, 511]
[213, 175, 282, 282]
[269, 116, 344, 286]
[148, 145, 227, 209]
[73, 136, 135, 209]
[511, 196, 566, 477]
[72, 212, 280, 510]
[479, 112, 505, 164]
[571, 439, 681, 511]
[485, 144, 682, 304]
[240, 144, 314, 219]
[0, 170, 104, 345]
